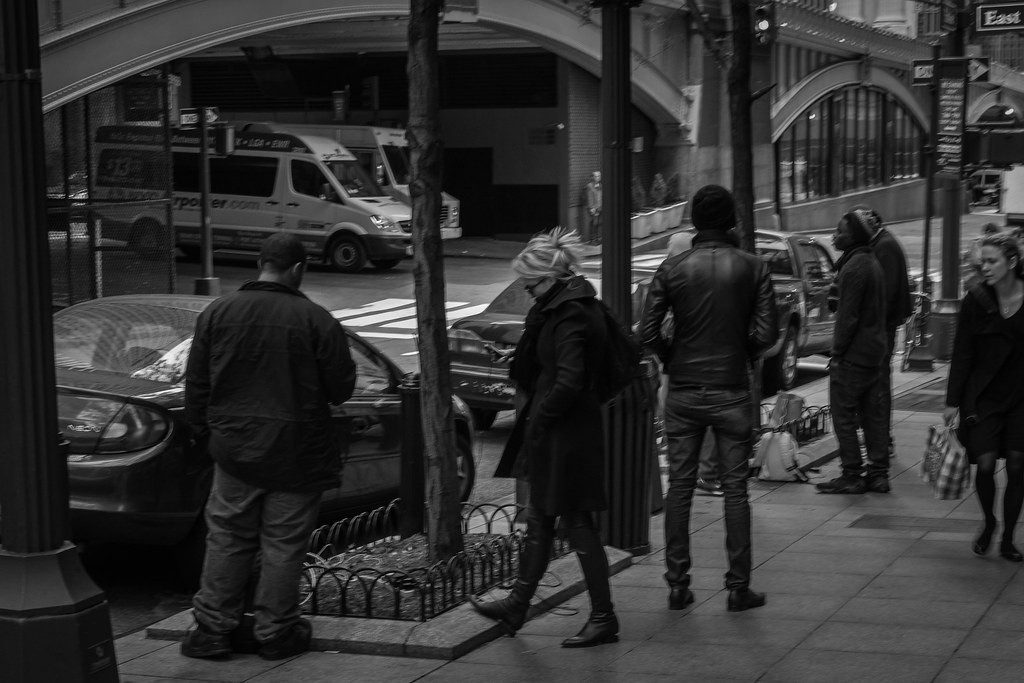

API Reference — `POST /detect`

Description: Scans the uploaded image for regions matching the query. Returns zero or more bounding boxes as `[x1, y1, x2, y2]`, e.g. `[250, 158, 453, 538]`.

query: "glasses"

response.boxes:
[524, 279, 546, 296]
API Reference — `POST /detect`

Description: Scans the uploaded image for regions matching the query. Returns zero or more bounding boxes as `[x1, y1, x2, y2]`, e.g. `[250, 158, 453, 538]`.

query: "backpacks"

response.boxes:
[752, 429, 809, 482]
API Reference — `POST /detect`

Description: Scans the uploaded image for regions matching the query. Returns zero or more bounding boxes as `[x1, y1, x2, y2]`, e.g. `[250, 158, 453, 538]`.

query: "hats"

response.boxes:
[691, 184, 736, 231]
[848, 209, 882, 242]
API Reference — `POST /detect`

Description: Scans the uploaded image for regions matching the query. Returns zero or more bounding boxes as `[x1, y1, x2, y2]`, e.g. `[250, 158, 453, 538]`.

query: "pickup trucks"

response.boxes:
[688, 228, 838, 397]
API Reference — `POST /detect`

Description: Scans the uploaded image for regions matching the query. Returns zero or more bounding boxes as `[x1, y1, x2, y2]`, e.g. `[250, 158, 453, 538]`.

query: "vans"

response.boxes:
[87, 122, 414, 273]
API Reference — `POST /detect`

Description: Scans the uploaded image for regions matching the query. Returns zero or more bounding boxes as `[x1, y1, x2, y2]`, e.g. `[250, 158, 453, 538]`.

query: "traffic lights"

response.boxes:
[755, 3, 778, 47]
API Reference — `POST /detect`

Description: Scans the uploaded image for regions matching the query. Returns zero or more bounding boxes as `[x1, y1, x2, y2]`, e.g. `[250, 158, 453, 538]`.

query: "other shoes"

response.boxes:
[971, 523, 995, 552]
[1002, 544, 1023, 560]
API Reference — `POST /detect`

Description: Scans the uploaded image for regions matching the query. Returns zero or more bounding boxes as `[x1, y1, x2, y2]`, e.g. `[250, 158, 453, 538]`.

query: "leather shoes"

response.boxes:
[668, 588, 694, 610]
[727, 588, 766, 611]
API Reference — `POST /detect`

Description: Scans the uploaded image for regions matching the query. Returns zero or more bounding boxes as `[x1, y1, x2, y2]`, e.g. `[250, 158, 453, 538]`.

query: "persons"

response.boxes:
[641, 185, 778, 612]
[471, 229, 621, 648]
[586, 171, 603, 246]
[944, 222, 1024, 562]
[815, 204, 913, 493]
[179, 232, 357, 661]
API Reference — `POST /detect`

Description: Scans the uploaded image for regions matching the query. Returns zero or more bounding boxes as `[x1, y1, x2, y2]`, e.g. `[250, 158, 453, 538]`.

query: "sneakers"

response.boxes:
[258, 620, 311, 659]
[816, 474, 866, 494]
[863, 473, 889, 493]
[182, 625, 233, 658]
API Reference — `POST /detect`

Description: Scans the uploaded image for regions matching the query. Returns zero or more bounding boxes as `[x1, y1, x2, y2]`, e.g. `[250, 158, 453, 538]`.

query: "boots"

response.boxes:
[471, 514, 554, 637]
[559, 522, 619, 647]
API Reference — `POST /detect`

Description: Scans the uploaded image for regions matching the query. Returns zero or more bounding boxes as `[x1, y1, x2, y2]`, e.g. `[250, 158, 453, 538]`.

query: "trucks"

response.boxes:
[229, 121, 462, 260]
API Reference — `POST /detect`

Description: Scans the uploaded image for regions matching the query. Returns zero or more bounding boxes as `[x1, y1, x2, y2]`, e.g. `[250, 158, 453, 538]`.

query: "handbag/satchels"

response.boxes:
[921, 411, 969, 500]
[599, 300, 640, 401]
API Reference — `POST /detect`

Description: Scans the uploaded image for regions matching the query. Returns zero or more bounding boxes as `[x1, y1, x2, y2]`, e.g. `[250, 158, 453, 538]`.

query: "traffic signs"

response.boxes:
[913, 57, 989, 86]
[179, 107, 221, 127]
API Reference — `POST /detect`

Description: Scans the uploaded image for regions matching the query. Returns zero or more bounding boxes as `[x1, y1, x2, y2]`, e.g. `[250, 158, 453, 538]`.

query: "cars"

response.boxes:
[53, 294, 476, 590]
[969, 169, 1003, 206]
[448, 265, 663, 431]
[48, 171, 89, 205]
